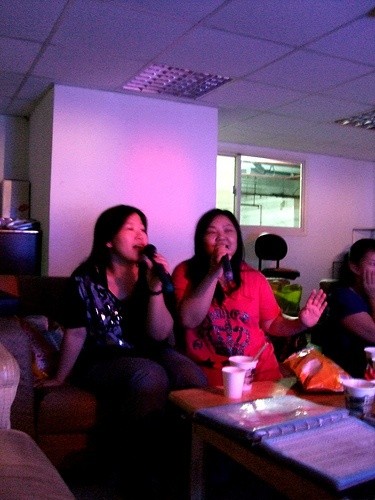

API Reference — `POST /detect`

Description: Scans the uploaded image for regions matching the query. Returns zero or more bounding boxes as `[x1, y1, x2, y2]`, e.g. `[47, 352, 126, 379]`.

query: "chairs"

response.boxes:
[255, 233, 300, 279]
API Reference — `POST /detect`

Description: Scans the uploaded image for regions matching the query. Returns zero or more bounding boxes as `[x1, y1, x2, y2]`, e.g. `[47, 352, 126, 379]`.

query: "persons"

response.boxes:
[323, 238, 375, 378]
[30, 204, 207, 500]
[172, 209, 327, 386]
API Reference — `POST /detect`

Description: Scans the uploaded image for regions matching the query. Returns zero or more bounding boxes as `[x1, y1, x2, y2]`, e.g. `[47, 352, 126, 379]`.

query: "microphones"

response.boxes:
[142, 244, 174, 293]
[222, 253, 233, 282]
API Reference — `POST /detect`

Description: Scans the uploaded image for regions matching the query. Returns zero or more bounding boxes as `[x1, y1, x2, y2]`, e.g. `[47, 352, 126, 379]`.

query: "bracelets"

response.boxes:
[150, 290, 161, 295]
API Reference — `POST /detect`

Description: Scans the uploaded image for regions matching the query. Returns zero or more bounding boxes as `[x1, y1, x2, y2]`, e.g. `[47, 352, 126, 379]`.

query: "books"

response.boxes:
[195, 394, 375, 492]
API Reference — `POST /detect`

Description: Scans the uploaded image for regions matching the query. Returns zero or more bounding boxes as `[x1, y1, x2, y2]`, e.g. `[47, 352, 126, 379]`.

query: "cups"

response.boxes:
[342, 378, 375, 417]
[222, 366, 246, 399]
[364, 346, 375, 380]
[229, 355, 258, 390]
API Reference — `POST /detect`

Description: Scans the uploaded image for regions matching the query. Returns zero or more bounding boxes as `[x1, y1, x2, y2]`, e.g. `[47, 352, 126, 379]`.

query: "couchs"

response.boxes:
[0, 317, 96, 500]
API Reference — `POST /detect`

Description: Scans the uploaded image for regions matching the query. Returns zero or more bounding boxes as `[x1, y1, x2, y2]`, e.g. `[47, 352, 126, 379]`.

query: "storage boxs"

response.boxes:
[1, 230, 39, 275]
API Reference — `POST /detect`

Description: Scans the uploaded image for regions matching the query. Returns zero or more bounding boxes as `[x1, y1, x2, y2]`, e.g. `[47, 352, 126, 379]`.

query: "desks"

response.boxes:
[170, 374, 375, 500]
